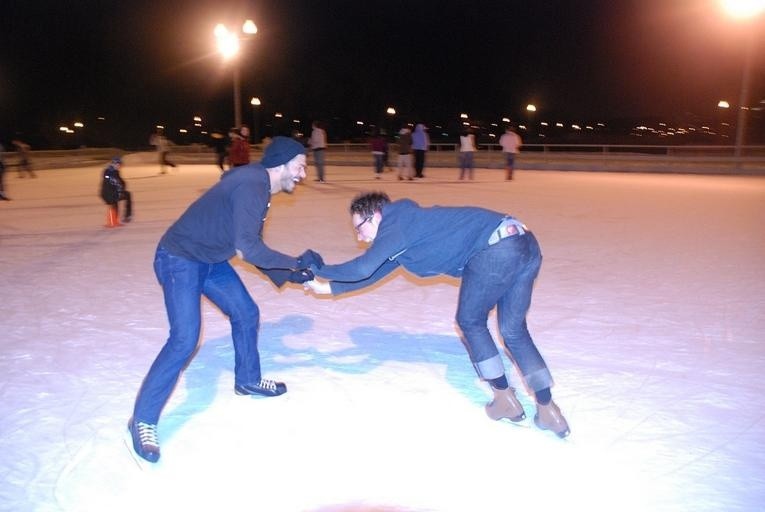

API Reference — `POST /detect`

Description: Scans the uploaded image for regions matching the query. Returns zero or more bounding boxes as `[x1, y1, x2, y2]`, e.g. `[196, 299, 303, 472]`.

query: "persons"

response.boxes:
[147, 125, 178, 174]
[127, 136, 323, 465]
[307, 120, 327, 183]
[10, 139, 38, 179]
[209, 124, 251, 180]
[97, 157, 133, 223]
[498, 126, 523, 181]
[370, 123, 431, 181]
[457, 128, 479, 180]
[303, 190, 571, 439]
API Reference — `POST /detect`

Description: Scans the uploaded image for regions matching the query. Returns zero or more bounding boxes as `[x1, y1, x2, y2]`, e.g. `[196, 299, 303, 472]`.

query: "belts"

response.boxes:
[489, 225, 529, 245]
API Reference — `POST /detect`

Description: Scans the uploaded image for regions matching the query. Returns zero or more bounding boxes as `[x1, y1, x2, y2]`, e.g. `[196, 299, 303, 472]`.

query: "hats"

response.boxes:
[261, 137, 306, 169]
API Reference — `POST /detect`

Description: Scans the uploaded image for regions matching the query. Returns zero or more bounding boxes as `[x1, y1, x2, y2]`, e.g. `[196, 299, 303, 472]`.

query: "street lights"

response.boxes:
[725, 0, 765, 169]
[214, 21, 258, 128]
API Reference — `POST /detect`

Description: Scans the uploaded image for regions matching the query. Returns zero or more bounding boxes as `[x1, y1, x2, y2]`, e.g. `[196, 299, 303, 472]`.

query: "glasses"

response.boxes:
[355, 218, 370, 234]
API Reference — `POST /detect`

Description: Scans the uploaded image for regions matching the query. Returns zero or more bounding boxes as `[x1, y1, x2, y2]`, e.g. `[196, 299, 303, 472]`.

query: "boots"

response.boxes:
[533, 401, 569, 439]
[485, 386, 527, 421]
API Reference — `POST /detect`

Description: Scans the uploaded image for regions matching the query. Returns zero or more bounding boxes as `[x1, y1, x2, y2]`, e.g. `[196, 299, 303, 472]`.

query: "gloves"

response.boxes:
[296, 249, 323, 273]
[288, 270, 312, 285]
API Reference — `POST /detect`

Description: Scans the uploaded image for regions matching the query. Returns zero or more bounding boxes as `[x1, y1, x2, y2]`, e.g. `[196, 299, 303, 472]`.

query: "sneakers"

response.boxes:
[127, 415, 159, 462]
[234, 379, 285, 397]
